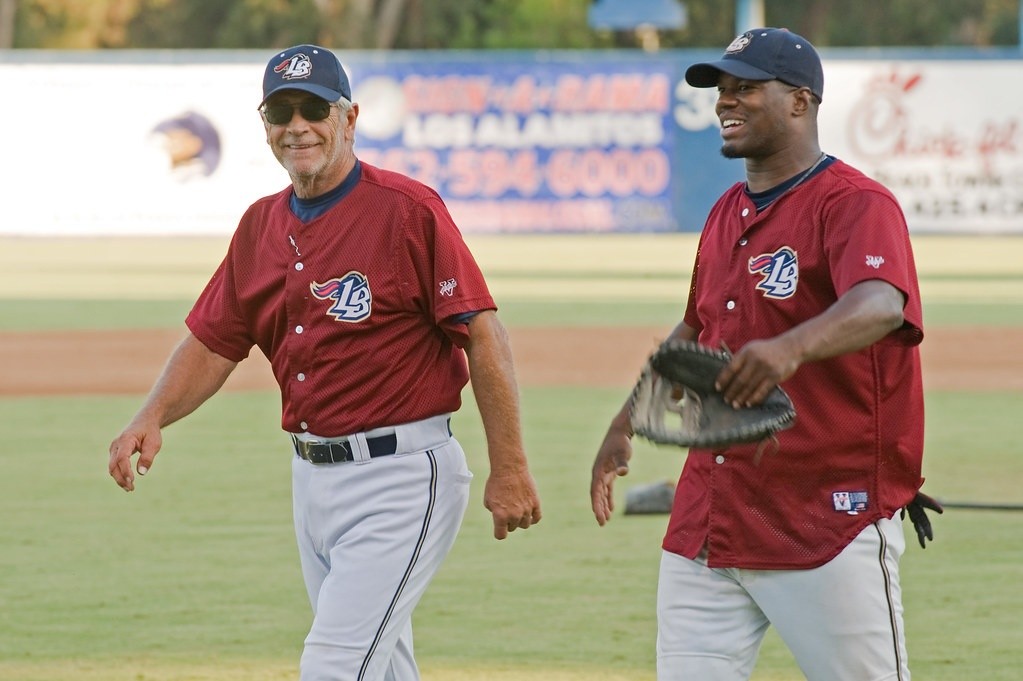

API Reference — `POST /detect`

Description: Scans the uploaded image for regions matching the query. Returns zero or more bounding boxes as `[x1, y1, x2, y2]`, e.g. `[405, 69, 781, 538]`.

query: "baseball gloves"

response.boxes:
[627, 338, 797, 447]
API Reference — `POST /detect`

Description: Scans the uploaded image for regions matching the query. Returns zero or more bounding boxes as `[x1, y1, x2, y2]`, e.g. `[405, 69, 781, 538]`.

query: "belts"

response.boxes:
[291, 417, 453, 464]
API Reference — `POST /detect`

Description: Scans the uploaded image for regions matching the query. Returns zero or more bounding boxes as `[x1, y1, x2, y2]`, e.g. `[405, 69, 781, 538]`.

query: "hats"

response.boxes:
[686, 28, 823, 103]
[258, 44, 351, 112]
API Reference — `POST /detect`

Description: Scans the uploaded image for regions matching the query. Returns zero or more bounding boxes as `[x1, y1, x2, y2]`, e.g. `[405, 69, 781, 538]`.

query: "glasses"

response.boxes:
[261, 97, 340, 126]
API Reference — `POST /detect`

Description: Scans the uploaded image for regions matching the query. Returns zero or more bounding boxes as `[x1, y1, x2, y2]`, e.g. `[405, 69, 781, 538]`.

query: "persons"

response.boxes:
[590, 27, 945, 681]
[106, 45, 542, 681]
[155, 118, 210, 184]
